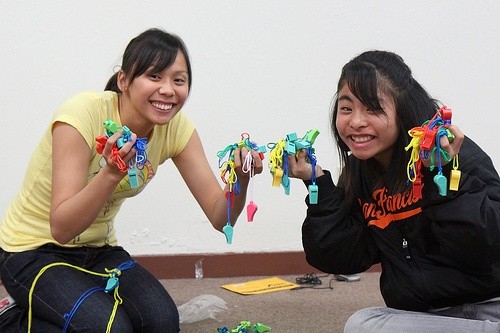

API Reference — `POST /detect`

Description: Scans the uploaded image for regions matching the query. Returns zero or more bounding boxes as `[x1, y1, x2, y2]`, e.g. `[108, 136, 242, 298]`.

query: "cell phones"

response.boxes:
[333, 274, 360, 282]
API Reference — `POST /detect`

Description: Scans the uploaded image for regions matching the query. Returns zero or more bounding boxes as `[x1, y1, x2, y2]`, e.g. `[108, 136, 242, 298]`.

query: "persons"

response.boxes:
[276, 50, 500, 333]
[0, 28, 263, 333]
